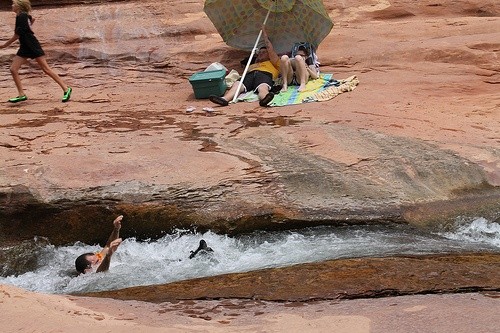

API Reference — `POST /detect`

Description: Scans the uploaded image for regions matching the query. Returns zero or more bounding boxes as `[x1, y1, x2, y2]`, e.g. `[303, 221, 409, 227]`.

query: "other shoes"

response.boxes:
[209, 95, 228, 106]
[259, 91, 274, 107]
[61, 86, 72, 102]
[9, 93, 27, 102]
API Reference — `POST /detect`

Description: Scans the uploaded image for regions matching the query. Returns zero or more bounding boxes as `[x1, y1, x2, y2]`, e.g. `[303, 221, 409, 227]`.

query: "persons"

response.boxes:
[281, 45, 317, 92]
[0, 0, 72, 104]
[74, 215, 215, 275]
[208, 23, 283, 106]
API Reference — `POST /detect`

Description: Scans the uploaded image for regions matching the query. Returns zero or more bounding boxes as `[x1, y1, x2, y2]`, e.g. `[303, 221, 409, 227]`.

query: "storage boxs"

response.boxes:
[188, 69, 226, 98]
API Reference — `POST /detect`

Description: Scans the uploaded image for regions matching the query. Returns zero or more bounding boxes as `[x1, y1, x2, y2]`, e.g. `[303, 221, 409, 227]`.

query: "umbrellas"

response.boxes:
[206, 0, 334, 103]
